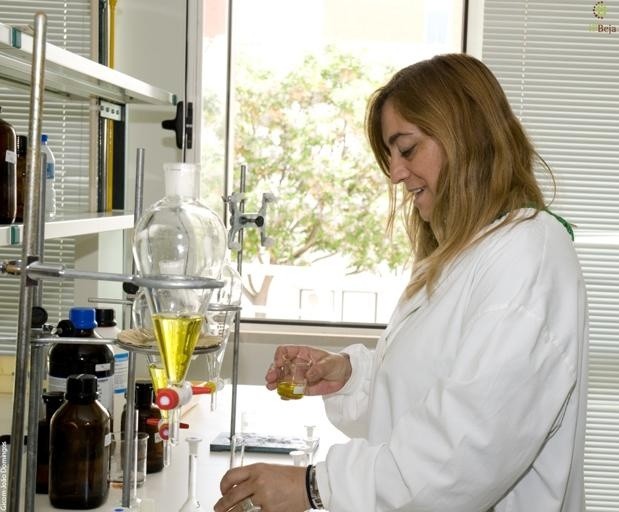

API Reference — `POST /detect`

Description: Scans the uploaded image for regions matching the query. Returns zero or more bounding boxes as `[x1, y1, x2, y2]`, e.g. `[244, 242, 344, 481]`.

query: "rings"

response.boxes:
[239, 497, 262, 512]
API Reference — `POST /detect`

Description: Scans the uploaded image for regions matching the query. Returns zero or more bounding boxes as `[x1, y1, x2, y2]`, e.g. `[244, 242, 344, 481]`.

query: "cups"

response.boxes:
[110, 430, 149, 489]
[277, 352, 313, 401]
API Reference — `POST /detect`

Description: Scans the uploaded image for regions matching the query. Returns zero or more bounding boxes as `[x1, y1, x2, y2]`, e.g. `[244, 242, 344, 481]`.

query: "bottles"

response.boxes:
[130, 187, 229, 447]
[196, 264, 244, 413]
[132, 285, 171, 469]
[120, 379, 165, 474]
[230, 433, 246, 488]
[40, 134, 57, 222]
[36, 301, 134, 511]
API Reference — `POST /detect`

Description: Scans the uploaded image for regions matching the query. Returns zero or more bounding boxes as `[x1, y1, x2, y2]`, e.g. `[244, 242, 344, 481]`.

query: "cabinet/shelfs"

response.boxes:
[0, 22, 177, 247]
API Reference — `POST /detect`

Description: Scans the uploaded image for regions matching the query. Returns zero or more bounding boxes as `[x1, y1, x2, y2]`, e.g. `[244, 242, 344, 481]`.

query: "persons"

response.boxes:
[214, 53, 589, 512]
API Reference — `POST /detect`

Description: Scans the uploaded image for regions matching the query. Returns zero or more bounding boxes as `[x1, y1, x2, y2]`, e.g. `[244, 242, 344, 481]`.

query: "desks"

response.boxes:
[34, 385, 348, 512]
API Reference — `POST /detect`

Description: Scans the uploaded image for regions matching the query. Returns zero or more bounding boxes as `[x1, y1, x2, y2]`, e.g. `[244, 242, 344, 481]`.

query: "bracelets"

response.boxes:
[306, 464, 324, 510]
